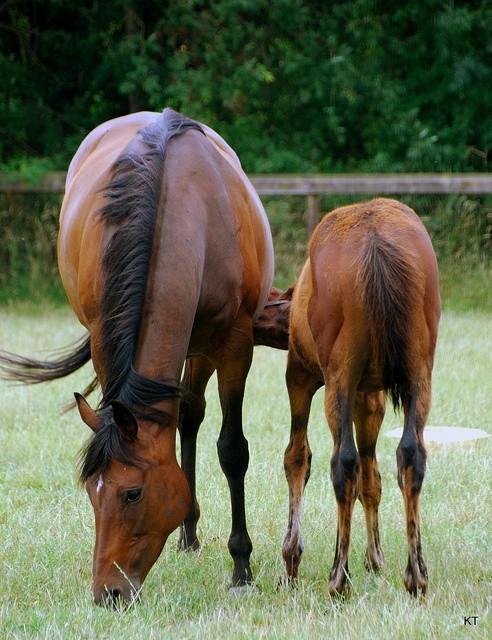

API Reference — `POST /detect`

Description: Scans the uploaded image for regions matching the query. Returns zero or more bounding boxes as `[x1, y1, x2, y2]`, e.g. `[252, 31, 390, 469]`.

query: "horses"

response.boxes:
[0, 108, 275, 609]
[253, 198, 441, 602]
